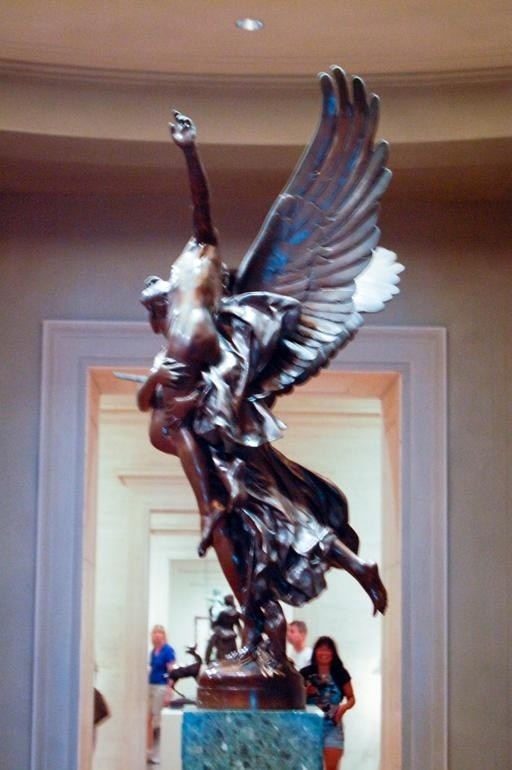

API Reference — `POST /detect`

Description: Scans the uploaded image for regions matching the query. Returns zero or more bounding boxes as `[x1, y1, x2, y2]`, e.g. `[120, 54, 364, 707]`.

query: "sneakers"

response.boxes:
[148, 757, 159, 764]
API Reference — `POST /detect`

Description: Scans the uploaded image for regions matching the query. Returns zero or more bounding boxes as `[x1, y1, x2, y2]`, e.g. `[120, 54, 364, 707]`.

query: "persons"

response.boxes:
[147, 624, 176, 763]
[287, 620, 314, 673]
[298, 635, 356, 770]
[93, 686, 110, 751]
[148, 110, 389, 618]
